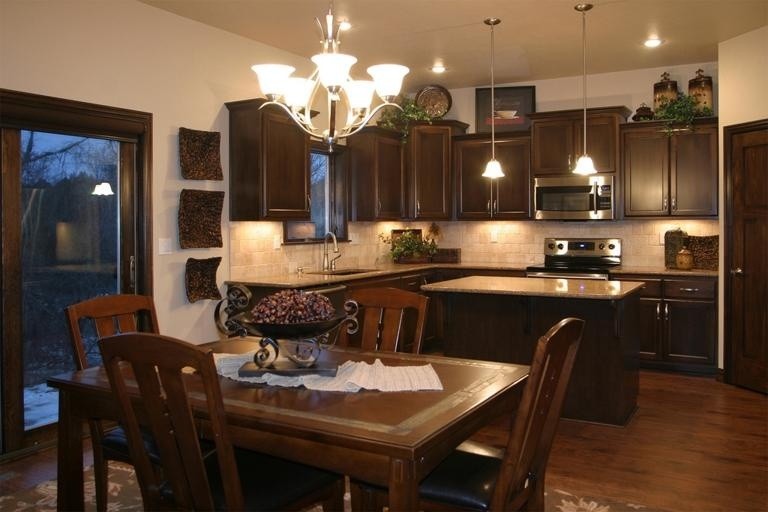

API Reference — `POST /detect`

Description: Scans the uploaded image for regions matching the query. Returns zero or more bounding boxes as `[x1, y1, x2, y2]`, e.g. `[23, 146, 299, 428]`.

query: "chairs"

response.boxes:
[345, 285, 430, 359]
[62, 289, 221, 511]
[346, 315, 595, 511]
[95, 325, 347, 510]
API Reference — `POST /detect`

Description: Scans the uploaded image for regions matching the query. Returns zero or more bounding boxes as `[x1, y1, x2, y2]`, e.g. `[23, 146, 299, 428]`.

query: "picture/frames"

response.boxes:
[473, 86, 536, 131]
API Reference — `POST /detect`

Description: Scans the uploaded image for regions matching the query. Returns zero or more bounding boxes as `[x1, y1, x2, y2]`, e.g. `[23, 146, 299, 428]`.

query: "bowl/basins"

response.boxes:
[230, 311, 347, 338]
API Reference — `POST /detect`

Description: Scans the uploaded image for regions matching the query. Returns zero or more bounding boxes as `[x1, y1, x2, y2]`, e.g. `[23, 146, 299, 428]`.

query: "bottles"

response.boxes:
[676, 244, 694, 270]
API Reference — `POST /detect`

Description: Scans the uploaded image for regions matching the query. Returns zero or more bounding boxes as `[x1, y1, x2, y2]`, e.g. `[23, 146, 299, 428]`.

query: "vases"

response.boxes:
[674, 245, 693, 271]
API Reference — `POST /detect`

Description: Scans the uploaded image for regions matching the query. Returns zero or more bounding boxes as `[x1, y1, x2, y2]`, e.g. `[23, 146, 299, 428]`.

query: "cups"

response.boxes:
[493, 110, 518, 118]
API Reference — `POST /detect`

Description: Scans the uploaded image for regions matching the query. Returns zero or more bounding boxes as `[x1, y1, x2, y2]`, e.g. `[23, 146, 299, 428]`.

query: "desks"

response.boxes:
[38, 332, 535, 510]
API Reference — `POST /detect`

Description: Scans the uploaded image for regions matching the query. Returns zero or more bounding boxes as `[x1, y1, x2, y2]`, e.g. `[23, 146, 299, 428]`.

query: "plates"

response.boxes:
[494, 117, 520, 120]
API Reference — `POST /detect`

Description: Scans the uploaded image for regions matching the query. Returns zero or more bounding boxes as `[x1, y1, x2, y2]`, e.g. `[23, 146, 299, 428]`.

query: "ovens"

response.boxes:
[527, 272, 609, 281]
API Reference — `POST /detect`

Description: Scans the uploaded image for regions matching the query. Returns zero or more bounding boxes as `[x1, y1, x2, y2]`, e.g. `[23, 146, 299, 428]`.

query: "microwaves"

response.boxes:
[531, 176, 616, 222]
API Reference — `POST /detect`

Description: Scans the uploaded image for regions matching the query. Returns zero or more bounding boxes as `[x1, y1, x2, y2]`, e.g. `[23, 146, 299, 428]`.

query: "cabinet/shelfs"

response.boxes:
[452, 130, 532, 223]
[438, 268, 524, 281]
[221, 99, 323, 224]
[524, 103, 632, 176]
[615, 117, 721, 219]
[349, 269, 439, 350]
[613, 272, 718, 378]
[227, 281, 350, 344]
[340, 121, 407, 223]
[406, 117, 470, 225]
[422, 280, 649, 431]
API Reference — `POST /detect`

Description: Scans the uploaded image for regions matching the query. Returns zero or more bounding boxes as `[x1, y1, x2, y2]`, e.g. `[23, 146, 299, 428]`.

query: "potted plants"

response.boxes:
[370, 226, 437, 266]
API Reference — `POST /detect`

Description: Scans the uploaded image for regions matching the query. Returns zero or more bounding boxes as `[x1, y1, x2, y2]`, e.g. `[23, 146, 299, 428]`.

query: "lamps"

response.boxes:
[481, 17, 506, 180]
[570, 2, 598, 179]
[244, 1, 412, 157]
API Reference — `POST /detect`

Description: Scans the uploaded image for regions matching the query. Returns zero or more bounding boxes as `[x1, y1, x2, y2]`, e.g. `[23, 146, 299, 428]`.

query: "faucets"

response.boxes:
[322, 230, 341, 273]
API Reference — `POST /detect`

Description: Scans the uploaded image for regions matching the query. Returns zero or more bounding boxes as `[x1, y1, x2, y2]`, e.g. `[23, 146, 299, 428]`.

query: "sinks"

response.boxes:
[305, 266, 386, 278]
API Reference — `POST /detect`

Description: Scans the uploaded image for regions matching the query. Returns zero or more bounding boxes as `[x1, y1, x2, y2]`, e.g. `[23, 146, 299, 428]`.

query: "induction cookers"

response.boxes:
[525, 257, 621, 273]
